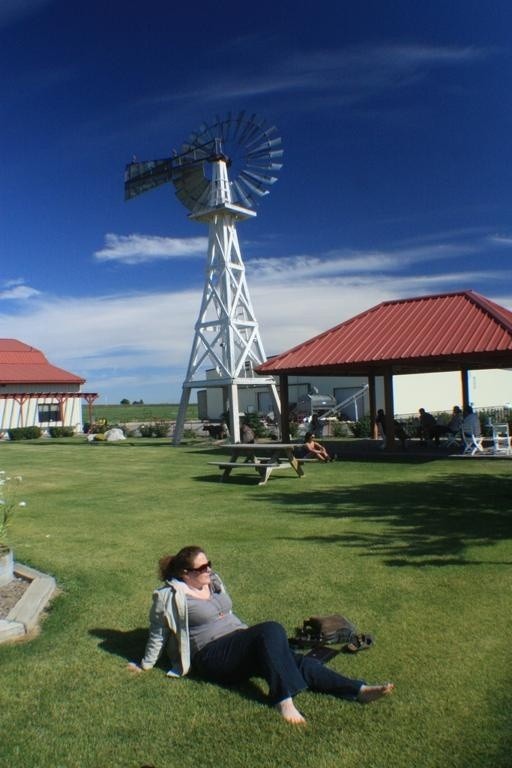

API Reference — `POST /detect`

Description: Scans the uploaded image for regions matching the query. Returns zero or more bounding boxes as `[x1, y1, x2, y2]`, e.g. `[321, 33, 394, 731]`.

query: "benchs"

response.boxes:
[255, 457, 318, 465]
[206, 461, 281, 470]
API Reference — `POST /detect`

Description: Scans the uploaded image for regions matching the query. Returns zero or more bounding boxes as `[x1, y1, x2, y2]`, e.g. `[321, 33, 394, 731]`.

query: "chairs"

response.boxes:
[376, 422, 512, 457]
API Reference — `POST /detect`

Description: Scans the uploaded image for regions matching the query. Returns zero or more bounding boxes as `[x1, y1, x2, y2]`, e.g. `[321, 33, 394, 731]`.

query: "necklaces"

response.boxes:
[190, 587, 225, 615]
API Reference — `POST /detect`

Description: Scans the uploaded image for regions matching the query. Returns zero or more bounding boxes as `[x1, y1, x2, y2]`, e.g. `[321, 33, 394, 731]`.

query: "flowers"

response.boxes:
[0, 470, 27, 552]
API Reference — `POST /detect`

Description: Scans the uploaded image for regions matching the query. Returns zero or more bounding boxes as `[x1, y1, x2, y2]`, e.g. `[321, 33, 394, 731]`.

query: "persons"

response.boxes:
[418, 406, 438, 442]
[120, 544, 396, 727]
[432, 405, 462, 446]
[458, 405, 482, 439]
[299, 431, 338, 464]
[374, 408, 410, 452]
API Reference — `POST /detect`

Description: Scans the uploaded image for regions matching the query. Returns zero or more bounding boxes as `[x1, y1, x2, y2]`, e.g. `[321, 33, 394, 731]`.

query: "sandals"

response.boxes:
[348, 633, 372, 653]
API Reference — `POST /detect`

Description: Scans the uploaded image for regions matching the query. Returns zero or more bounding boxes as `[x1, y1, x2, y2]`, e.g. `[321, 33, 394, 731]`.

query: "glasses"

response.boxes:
[183, 560, 212, 574]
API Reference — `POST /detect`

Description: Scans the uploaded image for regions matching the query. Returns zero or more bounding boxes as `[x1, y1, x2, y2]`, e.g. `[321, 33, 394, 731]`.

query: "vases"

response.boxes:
[0, 548, 15, 587]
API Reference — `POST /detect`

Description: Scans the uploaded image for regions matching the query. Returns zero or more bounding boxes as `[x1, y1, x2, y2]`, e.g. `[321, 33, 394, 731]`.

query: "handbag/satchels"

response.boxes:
[287, 613, 353, 650]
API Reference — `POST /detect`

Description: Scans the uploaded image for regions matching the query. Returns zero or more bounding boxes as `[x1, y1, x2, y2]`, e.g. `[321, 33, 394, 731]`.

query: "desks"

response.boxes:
[217, 443, 308, 485]
